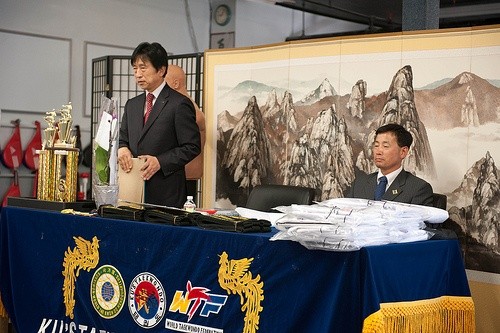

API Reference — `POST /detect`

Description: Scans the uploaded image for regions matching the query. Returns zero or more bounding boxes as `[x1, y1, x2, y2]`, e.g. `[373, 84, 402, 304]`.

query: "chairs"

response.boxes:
[246, 185, 315, 213]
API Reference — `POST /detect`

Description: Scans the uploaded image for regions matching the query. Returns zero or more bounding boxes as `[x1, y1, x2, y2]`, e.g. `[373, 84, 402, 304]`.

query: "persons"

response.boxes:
[118, 43, 200, 210]
[164, 65, 207, 208]
[346, 124, 435, 206]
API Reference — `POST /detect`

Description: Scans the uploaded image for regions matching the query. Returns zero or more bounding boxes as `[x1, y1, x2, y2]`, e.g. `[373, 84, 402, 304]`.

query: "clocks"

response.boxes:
[214, 4, 231, 26]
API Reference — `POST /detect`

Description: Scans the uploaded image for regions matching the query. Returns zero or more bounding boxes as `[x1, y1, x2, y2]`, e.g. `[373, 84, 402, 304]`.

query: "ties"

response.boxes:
[374, 176, 387, 201]
[143, 93, 154, 128]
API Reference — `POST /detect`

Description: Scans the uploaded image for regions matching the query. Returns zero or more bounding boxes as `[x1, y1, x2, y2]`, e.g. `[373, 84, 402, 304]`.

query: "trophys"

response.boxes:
[51, 104, 79, 202]
[35, 108, 61, 201]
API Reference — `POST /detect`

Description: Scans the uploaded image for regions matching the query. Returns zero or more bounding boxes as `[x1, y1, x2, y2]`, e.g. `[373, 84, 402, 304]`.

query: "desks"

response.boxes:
[0, 206, 475, 333]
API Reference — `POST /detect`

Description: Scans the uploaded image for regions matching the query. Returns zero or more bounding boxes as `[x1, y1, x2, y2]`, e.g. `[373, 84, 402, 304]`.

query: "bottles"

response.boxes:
[183, 196, 196, 211]
